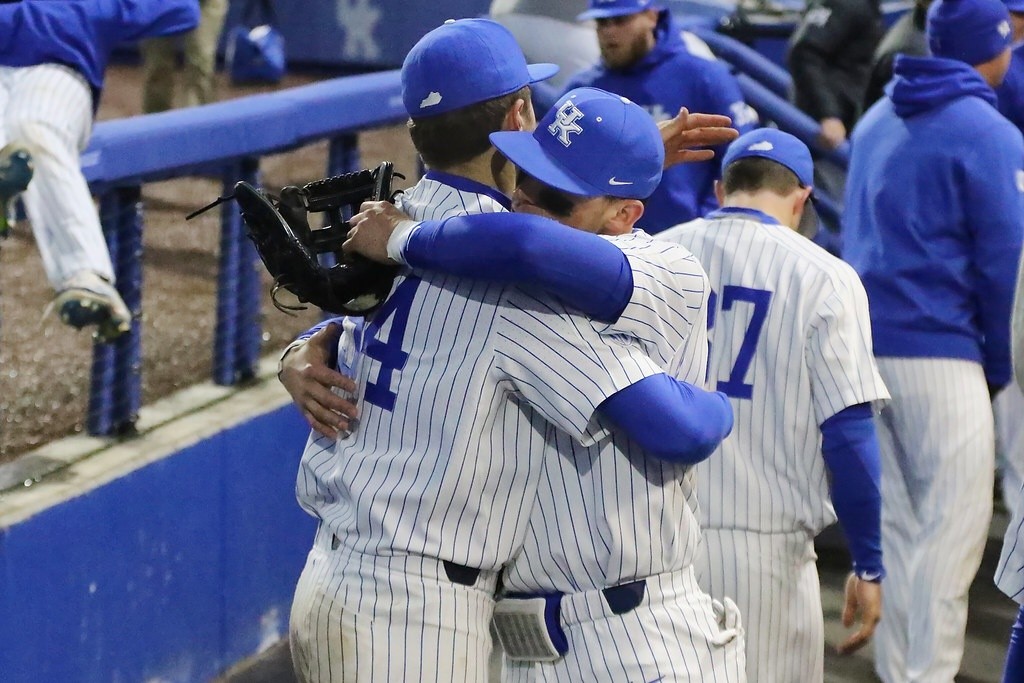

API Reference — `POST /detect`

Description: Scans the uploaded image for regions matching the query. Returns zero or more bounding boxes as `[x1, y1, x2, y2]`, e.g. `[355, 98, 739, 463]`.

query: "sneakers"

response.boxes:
[54, 274, 131, 343]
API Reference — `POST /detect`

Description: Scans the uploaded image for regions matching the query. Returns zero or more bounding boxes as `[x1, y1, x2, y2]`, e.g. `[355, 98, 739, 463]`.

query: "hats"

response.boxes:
[489, 86, 665, 199]
[927, 0, 1014, 64]
[1002, 0, 1024, 11]
[575, 0, 652, 20]
[400, 18, 561, 118]
[722, 128, 820, 201]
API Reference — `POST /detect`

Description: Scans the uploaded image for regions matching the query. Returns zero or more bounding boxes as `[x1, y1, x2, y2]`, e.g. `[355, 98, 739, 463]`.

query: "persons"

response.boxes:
[275, 0, 1024, 682]
[0, 0, 233, 347]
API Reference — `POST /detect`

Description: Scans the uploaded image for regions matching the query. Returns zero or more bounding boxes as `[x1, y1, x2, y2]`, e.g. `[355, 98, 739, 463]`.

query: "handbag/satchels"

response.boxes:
[225, 0, 285, 88]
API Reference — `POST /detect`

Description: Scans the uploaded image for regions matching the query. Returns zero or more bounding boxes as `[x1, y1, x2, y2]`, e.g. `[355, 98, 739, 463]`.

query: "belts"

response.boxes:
[332, 533, 481, 586]
[602, 579, 645, 615]
